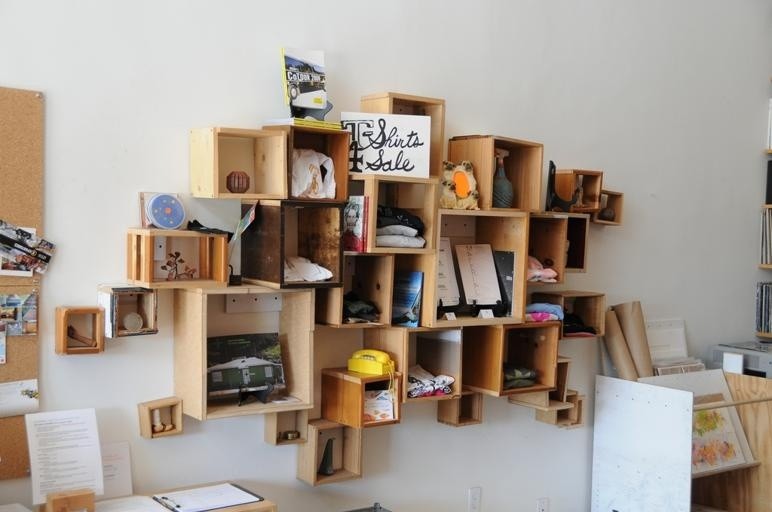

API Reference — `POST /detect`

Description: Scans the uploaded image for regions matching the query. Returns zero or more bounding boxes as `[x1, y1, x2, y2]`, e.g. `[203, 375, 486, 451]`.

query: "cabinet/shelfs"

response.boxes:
[755, 148, 772, 340]
[53, 90, 626, 488]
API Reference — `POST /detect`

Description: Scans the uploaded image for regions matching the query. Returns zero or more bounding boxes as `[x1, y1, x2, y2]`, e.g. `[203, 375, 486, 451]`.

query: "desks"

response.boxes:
[38, 478, 277, 512]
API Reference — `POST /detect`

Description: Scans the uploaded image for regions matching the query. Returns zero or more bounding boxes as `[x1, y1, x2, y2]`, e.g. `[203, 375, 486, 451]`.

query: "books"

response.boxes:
[756, 160, 772, 333]
[363, 390, 394, 423]
[91, 483, 263, 512]
[344, 195, 370, 254]
[263, 48, 341, 131]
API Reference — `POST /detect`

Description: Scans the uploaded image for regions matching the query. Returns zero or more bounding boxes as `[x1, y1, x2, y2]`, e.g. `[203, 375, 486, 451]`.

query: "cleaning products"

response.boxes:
[492, 147, 515, 208]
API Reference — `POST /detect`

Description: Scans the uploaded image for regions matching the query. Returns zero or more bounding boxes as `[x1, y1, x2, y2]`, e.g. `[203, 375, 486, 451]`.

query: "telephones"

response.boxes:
[348, 350, 395, 376]
